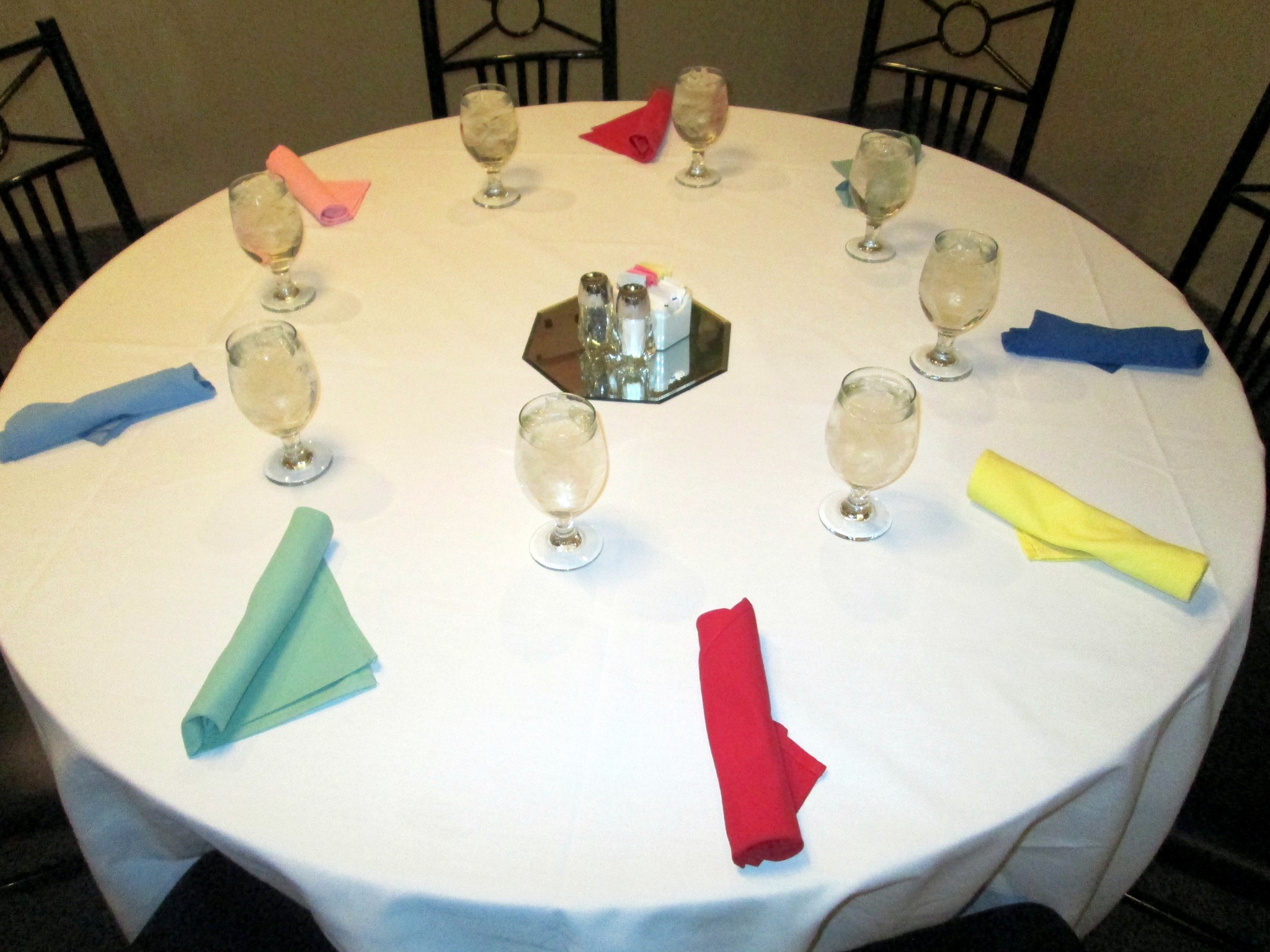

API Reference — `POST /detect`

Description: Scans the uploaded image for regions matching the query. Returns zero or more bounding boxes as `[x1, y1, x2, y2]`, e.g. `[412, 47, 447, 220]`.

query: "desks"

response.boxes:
[0, 101, 1269, 952]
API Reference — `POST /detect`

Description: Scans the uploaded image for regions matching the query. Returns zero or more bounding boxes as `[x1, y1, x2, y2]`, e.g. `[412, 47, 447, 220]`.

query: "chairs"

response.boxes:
[419, 0, 619, 120]
[1063, 85, 1269, 952]
[0, 17, 145, 387]
[849, 0, 1074, 179]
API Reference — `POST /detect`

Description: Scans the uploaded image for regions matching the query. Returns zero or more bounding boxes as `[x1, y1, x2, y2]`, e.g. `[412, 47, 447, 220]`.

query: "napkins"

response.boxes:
[580, 88, 673, 163]
[1002, 310, 1210, 374]
[697, 597, 827, 868]
[180, 506, 378, 758]
[266, 144, 371, 224]
[969, 449, 1210, 604]
[0, 363, 215, 462]
[832, 134, 920, 209]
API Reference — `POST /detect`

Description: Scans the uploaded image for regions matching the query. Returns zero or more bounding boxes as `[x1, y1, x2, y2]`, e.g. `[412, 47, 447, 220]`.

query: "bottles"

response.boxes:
[578, 271, 616, 353]
[616, 284, 658, 362]
[580, 351, 650, 402]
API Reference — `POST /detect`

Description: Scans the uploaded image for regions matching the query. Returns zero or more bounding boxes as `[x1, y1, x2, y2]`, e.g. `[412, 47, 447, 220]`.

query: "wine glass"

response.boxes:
[228, 172, 316, 313]
[672, 66, 728, 186]
[514, 392, 608, 570]
[846, 129, 915, 262]
[910, 228, 1001, 381]
[818, 366, 920, 541]
[460, 83, 521, 208]
[224, 320, 333, 487]
